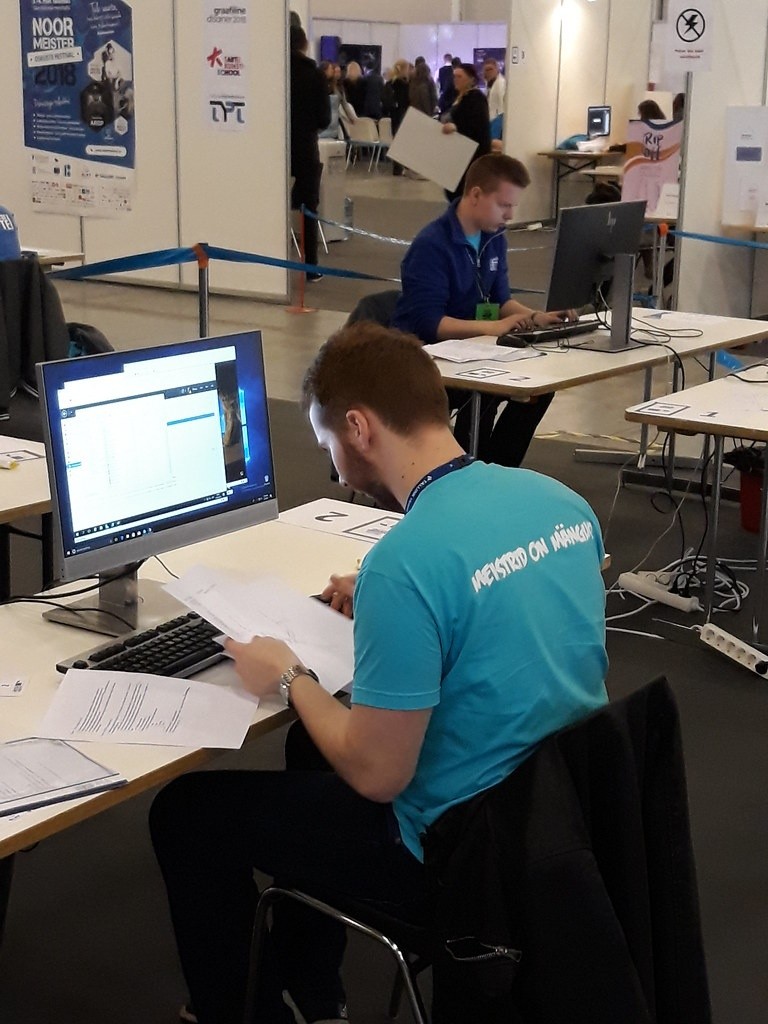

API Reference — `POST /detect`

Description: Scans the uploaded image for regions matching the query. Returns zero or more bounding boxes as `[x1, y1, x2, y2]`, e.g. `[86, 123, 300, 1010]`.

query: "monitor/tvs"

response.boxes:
[35, 329, 279, 636]
[545, 198, 647, 353]
[587, 105, 611, 140]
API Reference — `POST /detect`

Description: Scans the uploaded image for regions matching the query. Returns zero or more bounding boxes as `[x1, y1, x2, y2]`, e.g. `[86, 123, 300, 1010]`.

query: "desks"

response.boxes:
[39, 253, 84, 272]
[538, 150, 682, 228]
[434, 306, 768, 471]
[0, 497, 406, 939]
[0, 433, 53, 607]
[624, 361, 768, 655]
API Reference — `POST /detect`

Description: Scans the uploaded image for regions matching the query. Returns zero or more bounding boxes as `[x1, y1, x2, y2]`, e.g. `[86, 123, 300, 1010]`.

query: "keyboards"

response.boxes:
[512, 319, 605, 343]
[55, 610, 229, 678]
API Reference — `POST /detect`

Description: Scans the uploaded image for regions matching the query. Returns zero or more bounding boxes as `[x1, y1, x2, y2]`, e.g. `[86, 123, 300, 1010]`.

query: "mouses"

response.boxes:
[496, 332, 527, 348]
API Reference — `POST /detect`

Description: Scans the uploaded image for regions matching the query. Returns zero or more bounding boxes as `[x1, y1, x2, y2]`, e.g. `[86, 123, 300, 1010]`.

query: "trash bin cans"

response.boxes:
[738, 446, 768, 535]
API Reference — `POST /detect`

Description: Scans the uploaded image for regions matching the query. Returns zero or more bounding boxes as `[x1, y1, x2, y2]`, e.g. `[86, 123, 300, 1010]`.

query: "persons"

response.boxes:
[483, 57, 507, 140]
[390, 154, 578, 468]
[320, 54, 462, 176]
[441, 63, 492, 203]
[103, 44, 123, 91]
[289, 25, 332, 282]
[147, 320, 609, 1024]
[610, 100, 665, 152]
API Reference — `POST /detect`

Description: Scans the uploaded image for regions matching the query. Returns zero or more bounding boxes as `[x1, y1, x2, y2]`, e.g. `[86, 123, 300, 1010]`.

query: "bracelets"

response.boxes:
[531, 311, 542, 320]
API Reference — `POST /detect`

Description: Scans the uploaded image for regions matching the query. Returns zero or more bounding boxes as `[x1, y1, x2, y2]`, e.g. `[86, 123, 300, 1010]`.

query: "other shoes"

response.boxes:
[307, 271, 323, 282]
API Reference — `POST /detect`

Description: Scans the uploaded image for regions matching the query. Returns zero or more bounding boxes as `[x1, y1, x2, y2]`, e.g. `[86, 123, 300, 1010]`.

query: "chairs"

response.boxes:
[586, 181, 620, 205]
[0, 258, 115, 444]
[242, 671, 714, 1024]
[331, 290, 507, 507]
[338, 102, 394, 172]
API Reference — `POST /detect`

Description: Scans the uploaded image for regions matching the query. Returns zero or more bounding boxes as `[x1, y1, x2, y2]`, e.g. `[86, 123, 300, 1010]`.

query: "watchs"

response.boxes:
[279, 665, 319, 706]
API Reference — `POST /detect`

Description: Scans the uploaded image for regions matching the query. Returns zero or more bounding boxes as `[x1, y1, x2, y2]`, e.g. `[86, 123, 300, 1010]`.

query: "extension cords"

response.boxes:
[699, 622, 767, 681]
[619, 572, 699, 612]
